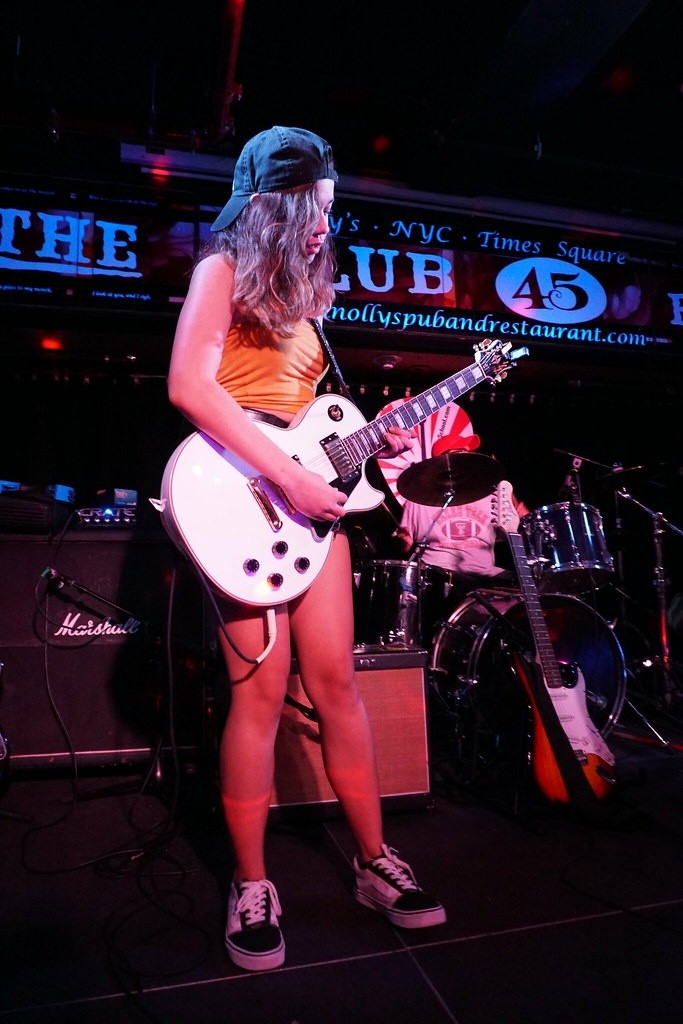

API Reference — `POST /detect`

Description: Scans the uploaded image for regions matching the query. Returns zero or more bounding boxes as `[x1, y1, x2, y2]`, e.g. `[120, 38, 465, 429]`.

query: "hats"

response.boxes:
[431, 434, 479, 457]
[209, 125, 340, 234]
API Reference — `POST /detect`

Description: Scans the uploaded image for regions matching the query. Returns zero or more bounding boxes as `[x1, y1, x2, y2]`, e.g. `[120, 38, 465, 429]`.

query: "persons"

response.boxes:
[168, 123, 444, 971]
[395, 430, 532, 618]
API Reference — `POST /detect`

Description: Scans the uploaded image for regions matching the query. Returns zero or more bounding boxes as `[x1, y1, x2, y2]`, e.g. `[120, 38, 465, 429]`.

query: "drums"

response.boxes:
[355, 559, 452, 653]
[428, 587, 627, 771]
[517, 501, 616, 596]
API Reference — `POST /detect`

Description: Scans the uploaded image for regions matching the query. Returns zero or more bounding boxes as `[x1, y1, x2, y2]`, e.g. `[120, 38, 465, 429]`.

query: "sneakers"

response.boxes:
[352, 840, 447, 930]
[224, 877, 287, 971]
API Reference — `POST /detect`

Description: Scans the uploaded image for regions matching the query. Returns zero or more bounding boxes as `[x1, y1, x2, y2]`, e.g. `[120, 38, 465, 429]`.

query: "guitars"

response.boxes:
[159, 339, 516, 608]
[489, 480, 619, 807]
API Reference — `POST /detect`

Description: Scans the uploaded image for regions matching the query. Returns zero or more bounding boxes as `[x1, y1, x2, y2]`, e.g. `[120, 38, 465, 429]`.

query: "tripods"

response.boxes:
[604, 474, 682, 712]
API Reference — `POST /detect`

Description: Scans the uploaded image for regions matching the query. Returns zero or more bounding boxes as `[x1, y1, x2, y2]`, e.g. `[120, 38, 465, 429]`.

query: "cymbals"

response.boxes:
[600, 462, 664, 482]
[396, 452, 506, 508]
[553, 446, 613, 470]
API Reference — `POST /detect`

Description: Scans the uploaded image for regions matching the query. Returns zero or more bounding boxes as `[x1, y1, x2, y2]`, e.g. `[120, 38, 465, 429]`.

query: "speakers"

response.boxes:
[268, 649, 433, 806]
[0, 526, 186, 772]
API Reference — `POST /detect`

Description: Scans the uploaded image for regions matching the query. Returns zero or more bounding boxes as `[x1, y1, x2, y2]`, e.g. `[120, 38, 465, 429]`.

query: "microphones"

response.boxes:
[558, 469, 579, 500]
[585, 690, 607, 711]
